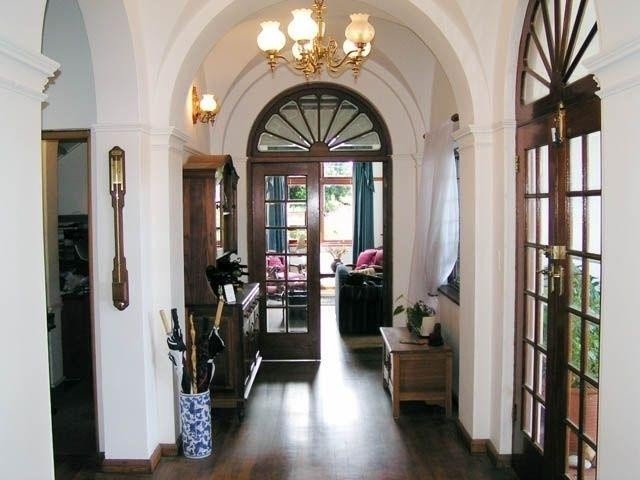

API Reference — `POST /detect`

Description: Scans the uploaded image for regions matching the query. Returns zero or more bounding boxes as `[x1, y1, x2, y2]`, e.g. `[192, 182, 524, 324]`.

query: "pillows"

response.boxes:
[269, 257, 282, 265]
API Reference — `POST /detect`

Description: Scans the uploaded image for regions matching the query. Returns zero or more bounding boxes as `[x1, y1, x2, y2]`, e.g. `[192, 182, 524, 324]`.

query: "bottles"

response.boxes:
[218, 262, 250, 279]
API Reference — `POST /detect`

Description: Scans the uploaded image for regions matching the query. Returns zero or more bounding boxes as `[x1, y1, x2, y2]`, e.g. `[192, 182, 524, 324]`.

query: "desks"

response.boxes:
[379, 327, 453, 422]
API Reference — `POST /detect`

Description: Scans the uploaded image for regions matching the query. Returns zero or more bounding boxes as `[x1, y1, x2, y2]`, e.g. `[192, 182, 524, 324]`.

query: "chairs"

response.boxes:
[266, 250, 307, 299]
[335, 246, 384, 334]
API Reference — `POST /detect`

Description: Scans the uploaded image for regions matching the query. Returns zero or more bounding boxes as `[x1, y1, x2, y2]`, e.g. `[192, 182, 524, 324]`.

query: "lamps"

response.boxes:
[192, 86, 220, 127]
[257, 0, 375, 83]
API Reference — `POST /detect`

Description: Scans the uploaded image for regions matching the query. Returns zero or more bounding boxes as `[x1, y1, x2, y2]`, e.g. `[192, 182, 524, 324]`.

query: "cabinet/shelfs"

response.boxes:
[184, 154, 263, 421]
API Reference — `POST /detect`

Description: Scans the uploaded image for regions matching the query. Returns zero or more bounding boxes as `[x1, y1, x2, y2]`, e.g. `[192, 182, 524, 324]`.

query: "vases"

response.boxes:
[331, 258, 343, 272]
[180, 388, 212, 459]
[288, 240, 298, 252]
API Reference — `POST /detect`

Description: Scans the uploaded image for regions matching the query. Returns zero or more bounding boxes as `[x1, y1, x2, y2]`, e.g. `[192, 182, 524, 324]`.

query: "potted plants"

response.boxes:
[394, 294, 440, 337]
[541, 262, 601, 455]
